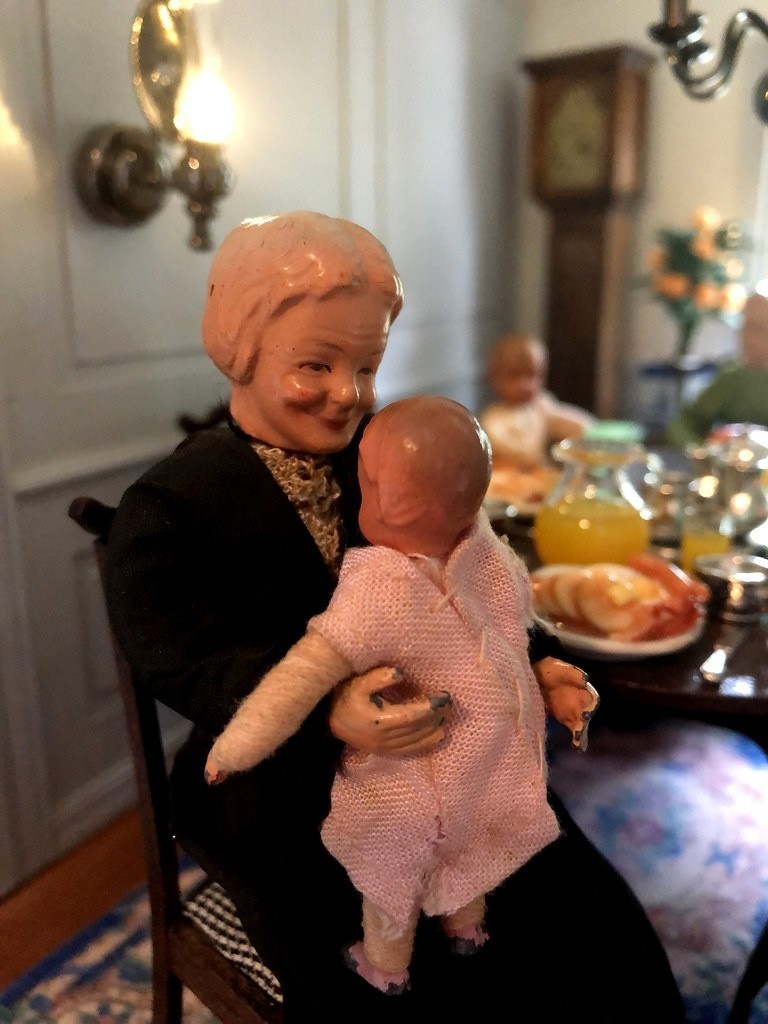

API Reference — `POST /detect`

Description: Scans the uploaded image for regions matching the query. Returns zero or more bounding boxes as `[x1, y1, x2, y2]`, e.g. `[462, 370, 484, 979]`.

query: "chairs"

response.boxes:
[70, 495, 292, 1024]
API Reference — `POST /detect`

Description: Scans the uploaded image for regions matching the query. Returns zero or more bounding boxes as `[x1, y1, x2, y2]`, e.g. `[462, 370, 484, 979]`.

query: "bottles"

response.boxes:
[536, 418, 668, 566]
[680, 423, 768, 577]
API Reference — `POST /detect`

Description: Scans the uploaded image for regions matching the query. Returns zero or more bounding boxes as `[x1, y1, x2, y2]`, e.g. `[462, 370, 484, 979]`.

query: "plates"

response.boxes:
[513, 577, 704, 658]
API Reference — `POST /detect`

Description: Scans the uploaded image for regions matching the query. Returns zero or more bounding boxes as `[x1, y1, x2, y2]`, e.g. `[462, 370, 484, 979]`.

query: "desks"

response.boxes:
[634, 359, 717, 445]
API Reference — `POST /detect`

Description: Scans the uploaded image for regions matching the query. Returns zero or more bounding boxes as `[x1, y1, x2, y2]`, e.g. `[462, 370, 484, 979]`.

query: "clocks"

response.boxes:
[511, 42, 657, 408]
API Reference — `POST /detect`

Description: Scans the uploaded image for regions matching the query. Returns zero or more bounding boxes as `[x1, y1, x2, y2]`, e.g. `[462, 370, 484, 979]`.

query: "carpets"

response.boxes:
[0, 723, 768, 1024]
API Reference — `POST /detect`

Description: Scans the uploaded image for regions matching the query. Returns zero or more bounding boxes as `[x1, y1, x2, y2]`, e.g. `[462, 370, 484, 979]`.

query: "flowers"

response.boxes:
[643, 214, 749, 341]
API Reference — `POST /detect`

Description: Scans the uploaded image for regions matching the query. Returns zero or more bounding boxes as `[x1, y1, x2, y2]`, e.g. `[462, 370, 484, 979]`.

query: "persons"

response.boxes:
[100, 209, 690, 1024]
[665, 294, 768, 455]
[479, 330, 596, 498]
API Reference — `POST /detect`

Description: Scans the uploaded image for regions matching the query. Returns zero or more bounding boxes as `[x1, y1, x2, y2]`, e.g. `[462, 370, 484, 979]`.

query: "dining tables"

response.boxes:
[477, 447, 767, 718]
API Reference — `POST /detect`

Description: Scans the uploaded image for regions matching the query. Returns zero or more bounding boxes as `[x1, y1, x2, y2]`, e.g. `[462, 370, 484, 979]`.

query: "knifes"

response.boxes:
[698, 627, 748, 684]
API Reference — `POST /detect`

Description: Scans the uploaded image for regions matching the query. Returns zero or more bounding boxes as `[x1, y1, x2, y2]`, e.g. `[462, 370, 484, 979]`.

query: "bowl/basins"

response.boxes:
[692, 553, 768, 622]
